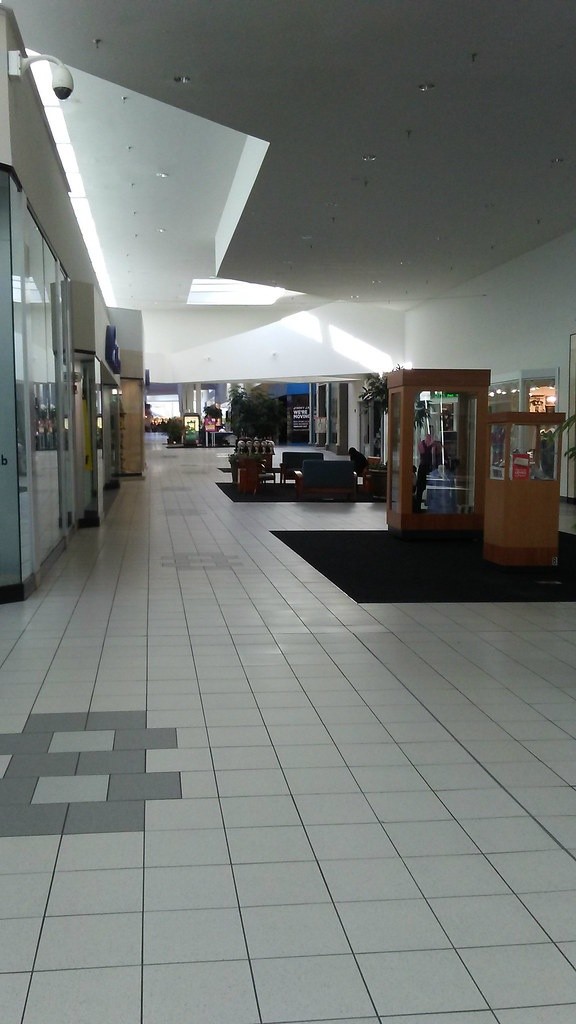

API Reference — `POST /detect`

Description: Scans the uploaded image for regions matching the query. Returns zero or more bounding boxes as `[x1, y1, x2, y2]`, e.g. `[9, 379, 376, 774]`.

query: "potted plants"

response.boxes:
[224, 385, 287, 495]
[161, 418, 182, 444]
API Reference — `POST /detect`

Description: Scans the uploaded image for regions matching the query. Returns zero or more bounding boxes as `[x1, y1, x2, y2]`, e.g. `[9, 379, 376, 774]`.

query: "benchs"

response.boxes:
[294, 460, 388, 501]
[280, 452, 324, 483]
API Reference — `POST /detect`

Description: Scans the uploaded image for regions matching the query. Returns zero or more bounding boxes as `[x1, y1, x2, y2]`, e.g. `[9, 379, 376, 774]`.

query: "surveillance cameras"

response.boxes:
[51, 65, 75, 100]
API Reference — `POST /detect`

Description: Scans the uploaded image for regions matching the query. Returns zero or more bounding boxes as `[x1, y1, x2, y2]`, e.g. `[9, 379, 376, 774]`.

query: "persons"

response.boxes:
[349, 447, 368, 477]
[416, 425, 442, 509]
[202, 413, 211, 447]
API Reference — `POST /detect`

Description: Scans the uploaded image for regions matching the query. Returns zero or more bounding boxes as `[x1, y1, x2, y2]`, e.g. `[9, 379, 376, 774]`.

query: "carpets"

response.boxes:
[269, 530, 576, 603]
[216, 482, 387, 503]
[218, 467, 282, 473]
